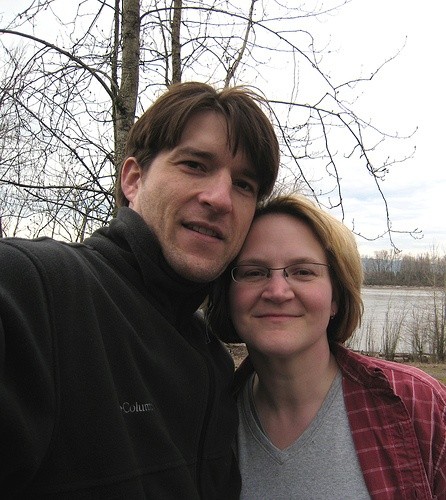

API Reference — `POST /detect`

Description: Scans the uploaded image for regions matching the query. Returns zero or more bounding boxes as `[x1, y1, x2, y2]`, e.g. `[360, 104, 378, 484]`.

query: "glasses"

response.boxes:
[230, 260, 329, 284]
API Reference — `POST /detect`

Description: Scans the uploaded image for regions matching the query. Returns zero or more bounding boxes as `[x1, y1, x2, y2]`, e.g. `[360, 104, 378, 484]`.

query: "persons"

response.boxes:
[201, 188, 446, 499]
[0, 79, 283, 499]
